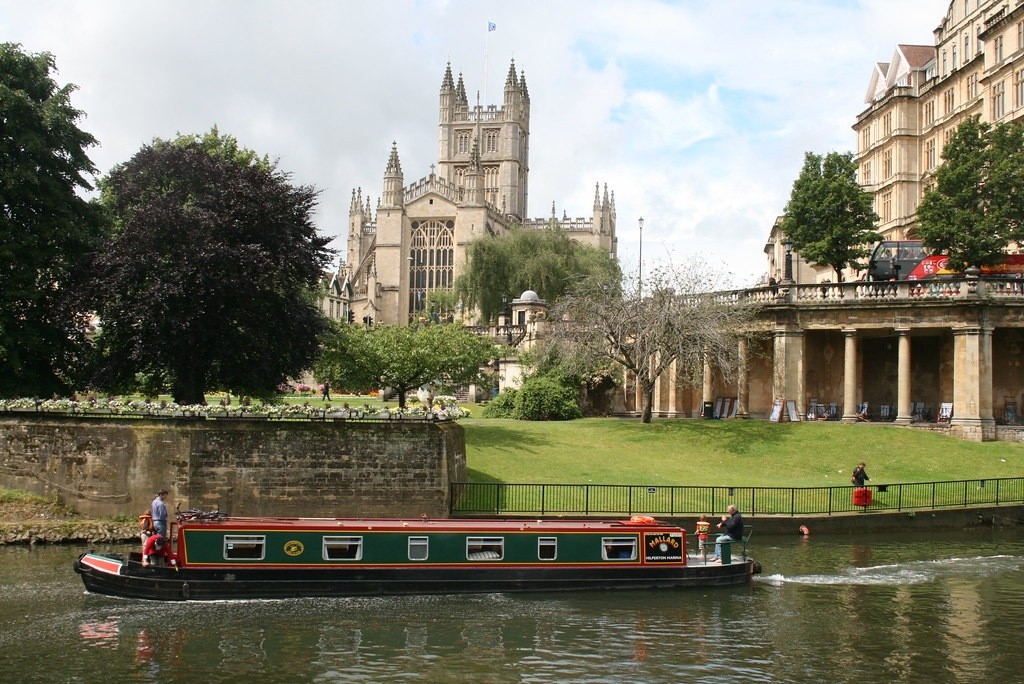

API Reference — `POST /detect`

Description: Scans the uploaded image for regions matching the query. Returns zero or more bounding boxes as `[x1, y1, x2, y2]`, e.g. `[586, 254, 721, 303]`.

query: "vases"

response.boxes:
[79, 409, 111, 413]
[184, 411, 227, 417]
[325, 412, 350, 418]
[0, 407, 7, 411]
[391, 414, 400, 419]
[228, 412, 241, 417]
[308, 412, 324, 418]
[159, 411, 183, 416]
[118, 410, 149, 414]
[242, 412, 268, 417]
[401, 413, 426, 420]
[149, 411, 154, 414]
[112, 411, 117, 413]
[270, 413, 281, 417]
[437, 414, 460, 420]
[282, 413, 308, 418]
[44, 408, 49, 412]
[37, 405, 44, 411]
[67, 407, 80, 413]
[48, 408, 67, 412]
[351, 412, 389, 420]
[427, 414, 435, 420]
[11, 407, 37, 411]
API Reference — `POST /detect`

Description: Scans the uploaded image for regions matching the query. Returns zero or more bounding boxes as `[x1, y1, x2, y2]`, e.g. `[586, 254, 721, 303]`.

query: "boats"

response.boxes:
[72, 514, 763, 602]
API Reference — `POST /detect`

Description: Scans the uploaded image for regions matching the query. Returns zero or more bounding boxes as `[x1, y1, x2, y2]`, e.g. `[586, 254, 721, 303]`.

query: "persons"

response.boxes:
[322, 379, 332, 401]
[770, 277, 783, 297]
[858, 402, 865, 413]
[141, 510, 153, 554]
[694, 515, 710, 562]
[821, 279, 828, 298]
[142, 534, 178, 572]
[151, 490, 167, 536]
[709, 504, 744, 564]
[851, 462, 872, 489]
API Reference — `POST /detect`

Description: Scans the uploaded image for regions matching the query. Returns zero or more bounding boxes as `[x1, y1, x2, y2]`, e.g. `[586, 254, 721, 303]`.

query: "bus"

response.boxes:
[867, 240, 1024, 294]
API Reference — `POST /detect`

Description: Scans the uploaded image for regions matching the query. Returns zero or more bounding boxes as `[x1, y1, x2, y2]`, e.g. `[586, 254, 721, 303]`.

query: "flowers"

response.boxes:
[0, 395, 471, 421]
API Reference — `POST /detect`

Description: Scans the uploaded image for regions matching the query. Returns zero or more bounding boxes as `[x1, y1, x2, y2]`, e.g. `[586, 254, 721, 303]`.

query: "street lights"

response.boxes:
[638, 215, 645, 301]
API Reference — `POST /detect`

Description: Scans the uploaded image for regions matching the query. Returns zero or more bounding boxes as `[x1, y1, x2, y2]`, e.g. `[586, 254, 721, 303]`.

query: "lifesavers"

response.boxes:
[631, 516, 655, 523]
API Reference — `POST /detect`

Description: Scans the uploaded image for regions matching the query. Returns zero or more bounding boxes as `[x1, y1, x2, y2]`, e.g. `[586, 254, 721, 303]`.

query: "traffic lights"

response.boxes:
[363, 316, 367, 325]
[369, 316, 373, 327]
[349, 309, 355, 324]
